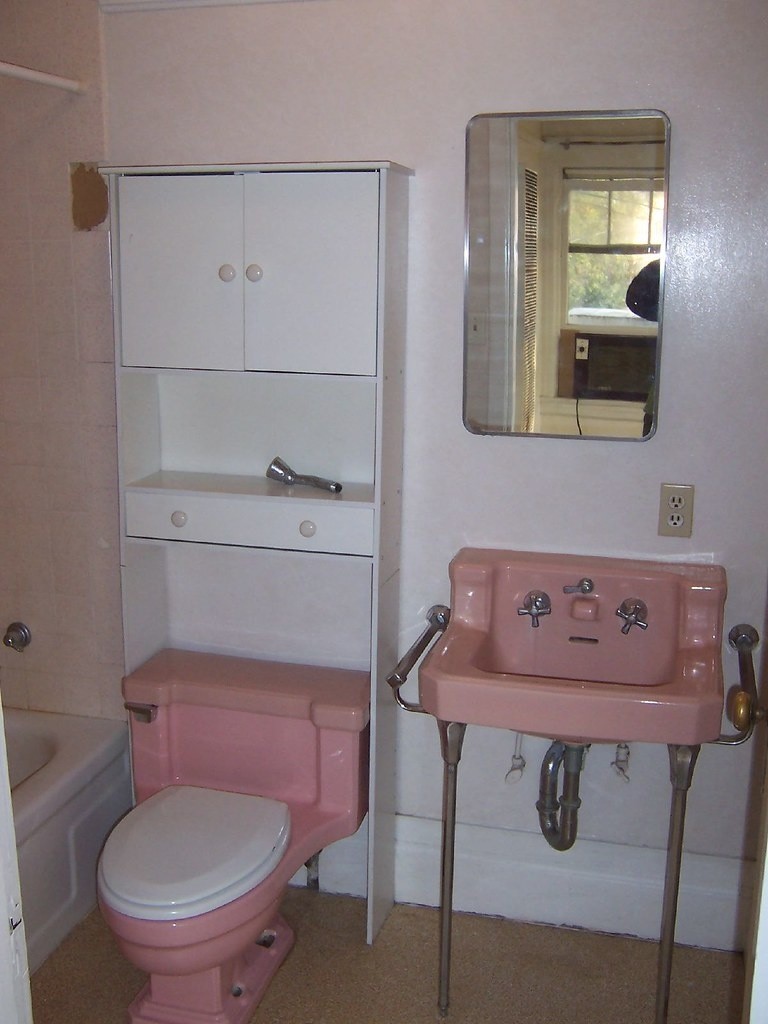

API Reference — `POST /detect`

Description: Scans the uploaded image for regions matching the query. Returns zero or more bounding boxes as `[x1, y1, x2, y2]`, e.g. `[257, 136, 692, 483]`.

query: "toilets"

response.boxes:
[95, 647, 374, 1024]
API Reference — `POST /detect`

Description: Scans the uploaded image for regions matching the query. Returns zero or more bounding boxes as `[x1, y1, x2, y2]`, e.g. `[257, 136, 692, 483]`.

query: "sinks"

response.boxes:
[417, 545, 729, 747]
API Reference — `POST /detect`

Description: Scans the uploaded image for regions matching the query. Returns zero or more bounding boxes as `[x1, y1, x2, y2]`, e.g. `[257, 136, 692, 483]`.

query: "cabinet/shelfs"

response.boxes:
[97, 160, 413, 946]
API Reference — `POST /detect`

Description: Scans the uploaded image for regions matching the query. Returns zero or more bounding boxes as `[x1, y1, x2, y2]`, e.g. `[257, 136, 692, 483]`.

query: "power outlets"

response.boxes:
[657, 483, 694, 537]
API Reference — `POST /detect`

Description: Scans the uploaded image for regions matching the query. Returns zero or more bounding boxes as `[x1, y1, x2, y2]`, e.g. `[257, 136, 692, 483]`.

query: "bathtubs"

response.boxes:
[0, 705, 129, 976]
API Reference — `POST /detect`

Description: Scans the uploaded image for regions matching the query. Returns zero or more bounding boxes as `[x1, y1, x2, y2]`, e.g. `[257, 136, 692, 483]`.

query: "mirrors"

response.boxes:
[462, 109, 671, 442]
